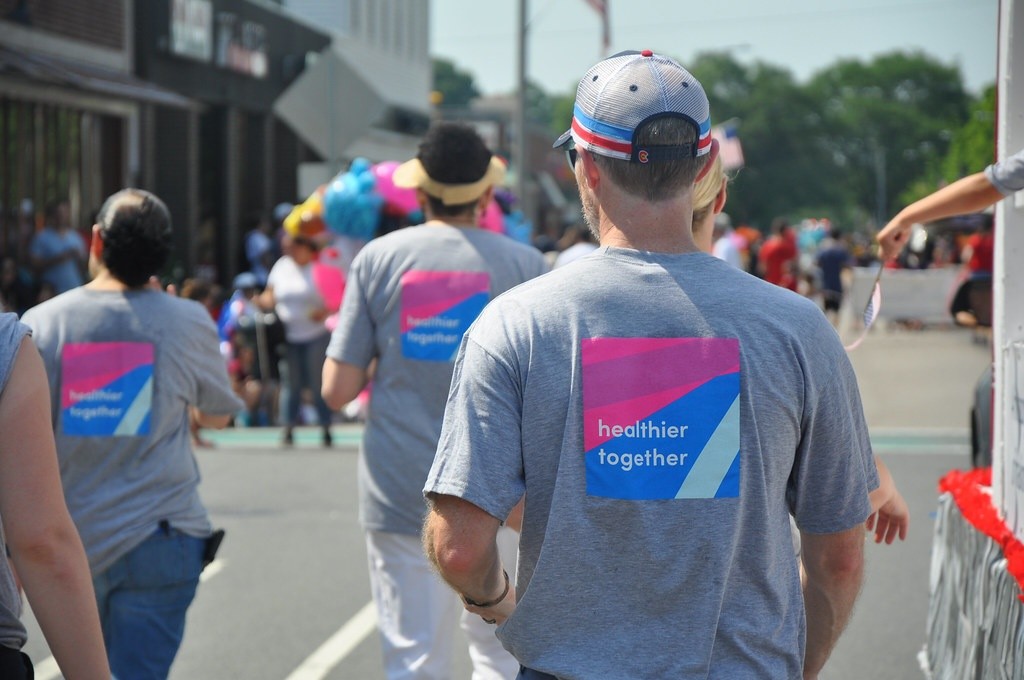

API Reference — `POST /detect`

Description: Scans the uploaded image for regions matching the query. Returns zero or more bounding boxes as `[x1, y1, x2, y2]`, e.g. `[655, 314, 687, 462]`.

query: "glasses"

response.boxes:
[565, 147, 596, 173]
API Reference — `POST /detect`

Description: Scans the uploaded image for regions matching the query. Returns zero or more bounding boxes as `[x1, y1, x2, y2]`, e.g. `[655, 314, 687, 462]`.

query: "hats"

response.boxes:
[391, 155, 507, 207]
[553, 49, 712, 162]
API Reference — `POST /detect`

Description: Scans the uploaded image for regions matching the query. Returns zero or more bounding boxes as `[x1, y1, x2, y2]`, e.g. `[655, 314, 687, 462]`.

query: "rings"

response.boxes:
[483, 618, 496, 624]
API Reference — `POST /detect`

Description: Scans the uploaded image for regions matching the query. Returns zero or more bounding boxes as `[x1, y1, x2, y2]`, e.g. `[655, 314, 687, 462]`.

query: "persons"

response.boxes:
[692, 153, 908, 559]
[714, 209, 993, 332]
[142, 234, 336, 446]
[876, 147, 1024, 259]
[316, 123, 548, 679]
[533, 226, 600, 271]
[18, 190, 239, 679]
[0, 310, 112, 680]
[0, 198, 98, 320]
[421, 49, 881, 680]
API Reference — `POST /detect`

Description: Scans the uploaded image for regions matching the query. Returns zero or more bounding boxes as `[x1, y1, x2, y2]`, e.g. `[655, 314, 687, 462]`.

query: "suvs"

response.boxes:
[948, 274, 994, 464]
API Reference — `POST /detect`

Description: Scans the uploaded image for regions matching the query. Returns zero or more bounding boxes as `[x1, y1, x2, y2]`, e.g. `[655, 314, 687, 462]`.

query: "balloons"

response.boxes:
[282, 150, 532, 406]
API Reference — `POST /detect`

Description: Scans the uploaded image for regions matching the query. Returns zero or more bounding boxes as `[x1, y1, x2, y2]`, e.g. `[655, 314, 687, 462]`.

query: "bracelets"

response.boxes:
[463, 570, 510, 608]
